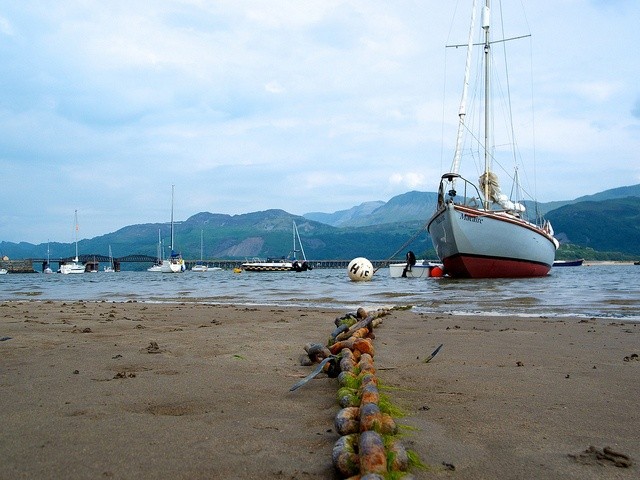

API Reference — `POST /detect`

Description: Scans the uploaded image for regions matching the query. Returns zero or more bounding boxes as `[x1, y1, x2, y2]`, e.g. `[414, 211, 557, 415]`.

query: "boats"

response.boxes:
[553, 259, 584, 266]
[426, 0, 558, 278]
[60, 264, 85, 275]
[243, 256, 302, 271]
[148, 264, 161, 272]
[161, 254, 186, 273]
[192, 265, 221, 272]
